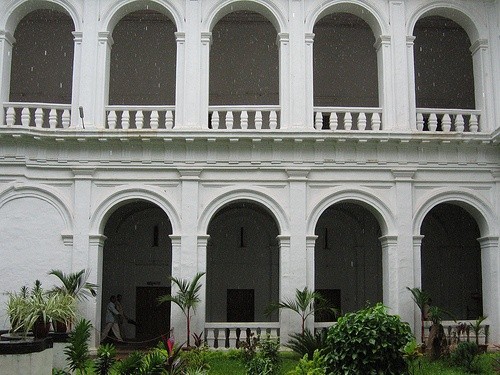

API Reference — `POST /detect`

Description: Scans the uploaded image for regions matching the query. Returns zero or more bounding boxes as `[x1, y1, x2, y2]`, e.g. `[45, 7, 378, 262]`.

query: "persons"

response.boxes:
[115, 293, 128, 342]
[100, 295, 128, 346]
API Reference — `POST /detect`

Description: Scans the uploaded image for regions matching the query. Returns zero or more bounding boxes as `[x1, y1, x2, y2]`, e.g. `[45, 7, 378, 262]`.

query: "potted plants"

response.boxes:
[466, 314, 489, 354]
[406, 286, 451, 362]
